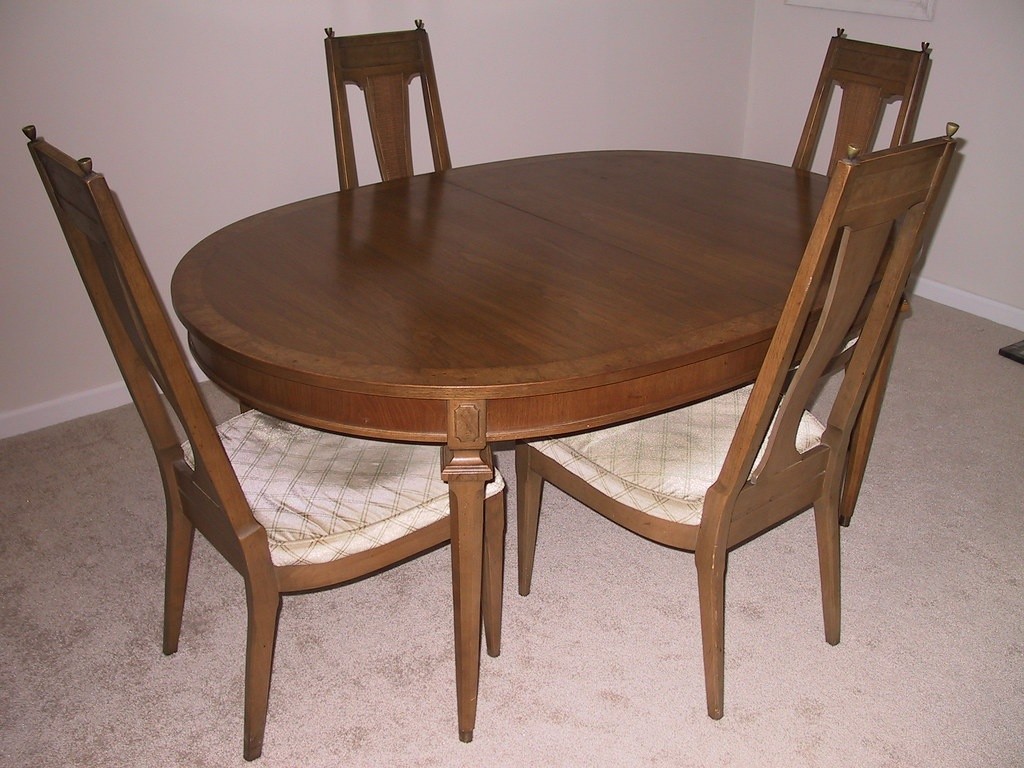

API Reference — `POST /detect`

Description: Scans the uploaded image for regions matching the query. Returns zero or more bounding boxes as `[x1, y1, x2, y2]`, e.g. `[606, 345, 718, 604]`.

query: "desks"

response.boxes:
[173, 151, 888, 741]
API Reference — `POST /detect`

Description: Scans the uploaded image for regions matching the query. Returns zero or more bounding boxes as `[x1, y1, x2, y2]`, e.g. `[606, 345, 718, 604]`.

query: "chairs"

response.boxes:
[515, 120, 964, 720]
[756, 22, 943, 411]
[322, 22, 451, 196]
[24, 124, 509, 760]
